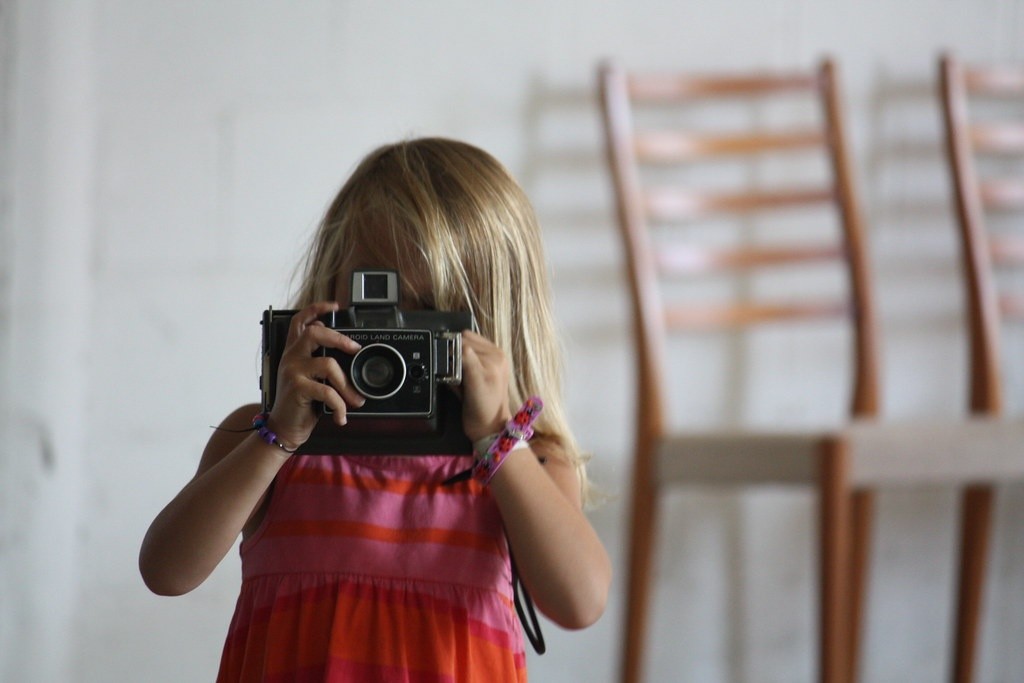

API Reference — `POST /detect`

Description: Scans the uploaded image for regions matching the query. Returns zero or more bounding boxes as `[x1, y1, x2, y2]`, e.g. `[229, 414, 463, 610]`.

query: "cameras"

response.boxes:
[310, 270, 463, 420]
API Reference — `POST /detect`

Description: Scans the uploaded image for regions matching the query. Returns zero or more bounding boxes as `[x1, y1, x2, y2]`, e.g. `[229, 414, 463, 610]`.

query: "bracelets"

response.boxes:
[473, 395, 543, 486]
[208, 391, 300, 453]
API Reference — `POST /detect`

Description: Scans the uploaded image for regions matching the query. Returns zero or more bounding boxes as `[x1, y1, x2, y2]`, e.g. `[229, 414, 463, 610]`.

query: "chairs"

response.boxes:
[597, 58, 1024, 682]
[939, 50, 1023, 683]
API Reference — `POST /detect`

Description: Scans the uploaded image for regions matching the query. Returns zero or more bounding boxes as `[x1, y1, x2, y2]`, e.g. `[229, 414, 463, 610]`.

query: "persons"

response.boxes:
[135, 138, 616, 683]
